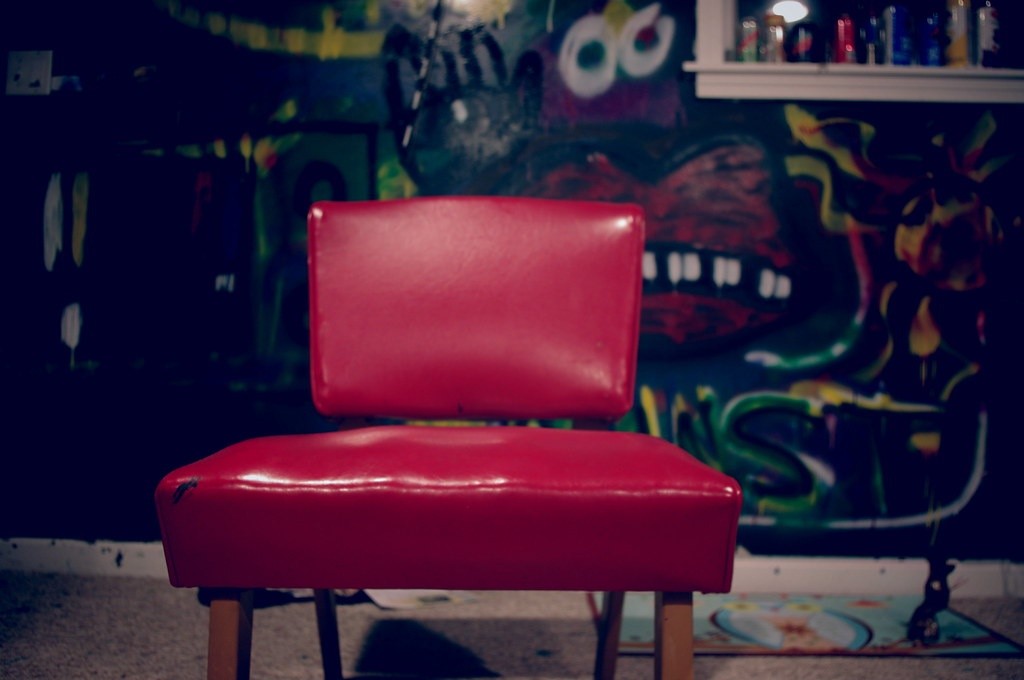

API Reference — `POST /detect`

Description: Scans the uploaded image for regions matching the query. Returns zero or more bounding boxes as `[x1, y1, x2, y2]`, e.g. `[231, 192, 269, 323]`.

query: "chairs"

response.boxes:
[151, 194, 744, 679]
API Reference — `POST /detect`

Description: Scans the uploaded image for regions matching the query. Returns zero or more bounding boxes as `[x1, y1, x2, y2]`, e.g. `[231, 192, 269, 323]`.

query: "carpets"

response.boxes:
[582, 589, 1024, 656]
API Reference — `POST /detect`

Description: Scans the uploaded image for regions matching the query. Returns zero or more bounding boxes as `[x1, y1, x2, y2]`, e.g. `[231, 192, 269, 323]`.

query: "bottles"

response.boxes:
[736, 0, 1014, 72]
